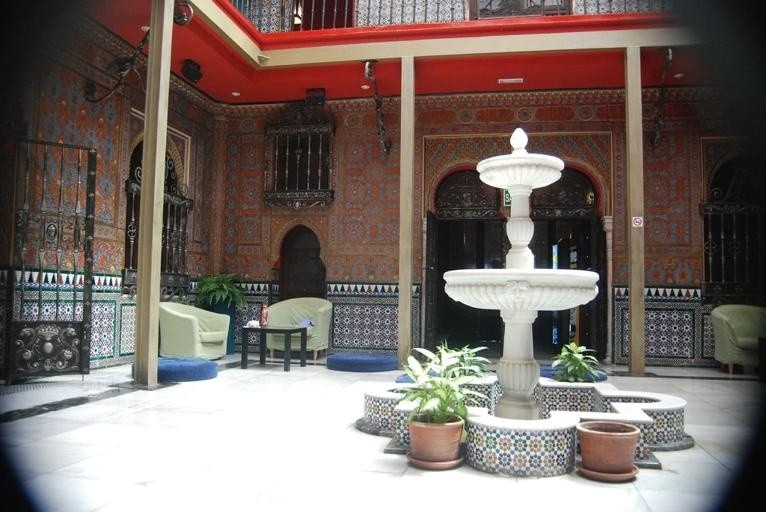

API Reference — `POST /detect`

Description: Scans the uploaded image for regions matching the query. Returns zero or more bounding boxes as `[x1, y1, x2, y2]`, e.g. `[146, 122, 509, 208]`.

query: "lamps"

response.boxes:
[651, 46, 680, 150]
[179, 57, 202, 82]
[84, 3, 194, 102]
[361, 59, 391, 155]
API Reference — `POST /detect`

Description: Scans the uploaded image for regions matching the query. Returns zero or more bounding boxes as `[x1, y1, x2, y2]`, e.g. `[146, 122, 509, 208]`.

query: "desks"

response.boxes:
[242, 324, 308, 371]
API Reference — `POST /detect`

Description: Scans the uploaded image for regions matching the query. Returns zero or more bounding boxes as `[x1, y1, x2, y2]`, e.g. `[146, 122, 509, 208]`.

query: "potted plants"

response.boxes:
[392, 377, 466, 469]
[198, 273, 246, 356]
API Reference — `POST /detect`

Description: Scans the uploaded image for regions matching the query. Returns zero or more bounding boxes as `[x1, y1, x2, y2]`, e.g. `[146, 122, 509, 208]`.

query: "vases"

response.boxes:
[578, 423, 639, 483]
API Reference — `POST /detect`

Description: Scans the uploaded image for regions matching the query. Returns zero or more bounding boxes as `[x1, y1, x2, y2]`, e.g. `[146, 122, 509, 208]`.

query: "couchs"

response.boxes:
[157, 298, 229, 361]
[264, 295, 334, 361]
[710, 301, 765, 377]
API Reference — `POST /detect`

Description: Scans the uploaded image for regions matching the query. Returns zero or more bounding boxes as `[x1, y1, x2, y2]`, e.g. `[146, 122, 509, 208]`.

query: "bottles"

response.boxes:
[261, 304, 268, 326]
